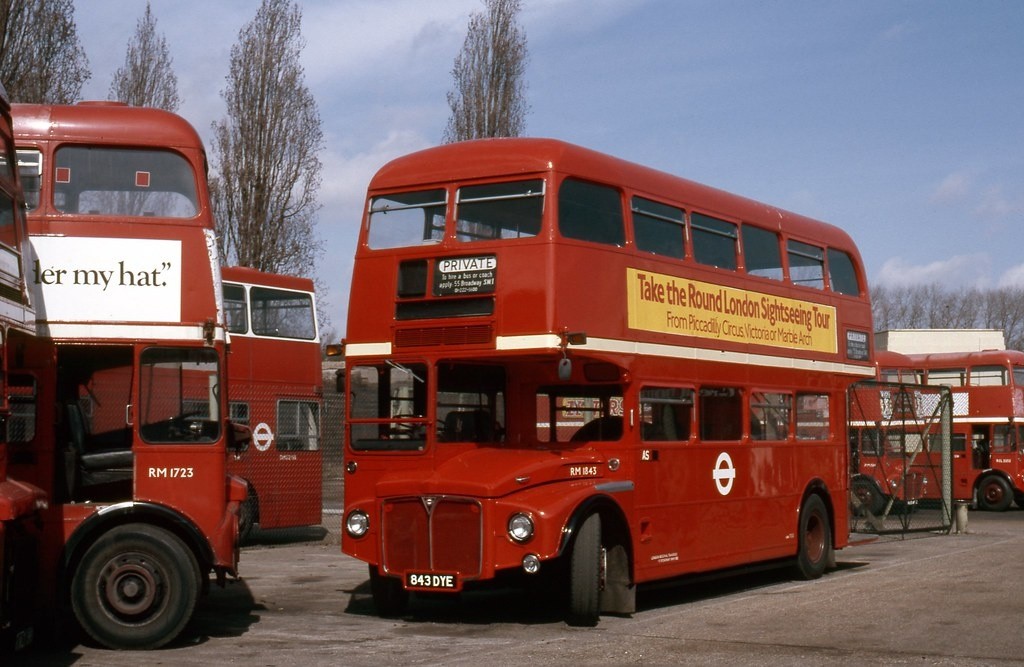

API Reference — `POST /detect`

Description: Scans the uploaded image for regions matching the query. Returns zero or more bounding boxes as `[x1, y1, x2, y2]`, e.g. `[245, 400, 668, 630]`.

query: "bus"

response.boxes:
[218, 263, 322, 546]
[6, 97, 257, 651]
[0, 84, 58, 666]
[537, 347, 1024, 516]
[323, 137, 877, 627]
